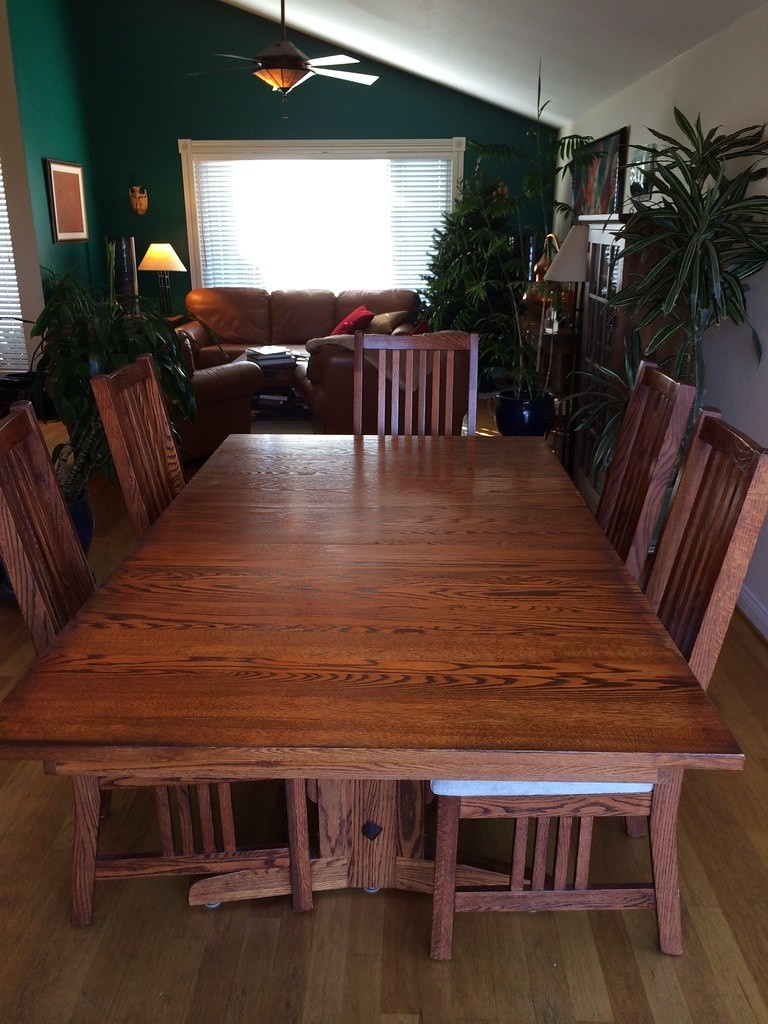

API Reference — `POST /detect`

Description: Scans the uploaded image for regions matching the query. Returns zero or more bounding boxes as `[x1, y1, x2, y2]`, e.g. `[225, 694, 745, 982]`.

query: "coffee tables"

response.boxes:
[232, 351, 312, 420]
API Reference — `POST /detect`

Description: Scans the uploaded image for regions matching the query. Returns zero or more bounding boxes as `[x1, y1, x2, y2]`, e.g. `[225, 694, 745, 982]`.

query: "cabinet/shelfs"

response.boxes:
[573, 213, 690, 495]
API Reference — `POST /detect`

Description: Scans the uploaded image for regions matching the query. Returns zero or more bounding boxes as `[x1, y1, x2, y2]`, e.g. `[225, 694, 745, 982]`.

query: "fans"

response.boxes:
[184, 0, 379, 85]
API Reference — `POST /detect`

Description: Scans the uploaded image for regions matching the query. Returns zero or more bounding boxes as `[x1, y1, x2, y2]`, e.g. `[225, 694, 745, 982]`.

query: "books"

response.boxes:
[246, 345, 296, 365]
[256, 390, 292, 405]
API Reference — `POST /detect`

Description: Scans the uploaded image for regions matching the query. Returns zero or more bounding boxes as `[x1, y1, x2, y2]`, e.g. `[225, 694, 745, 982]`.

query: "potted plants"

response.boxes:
[0, 234, 198, 605]
[409, 54, 768, 557]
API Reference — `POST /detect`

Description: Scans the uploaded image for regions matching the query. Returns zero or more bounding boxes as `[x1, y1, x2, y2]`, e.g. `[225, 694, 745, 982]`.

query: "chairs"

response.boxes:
[596, 359, 694, 578]
[353, 329, 478, 437]
[428, 407, 768, 959]
[89, 352, 185, 535]
[0, 399, 315, 927]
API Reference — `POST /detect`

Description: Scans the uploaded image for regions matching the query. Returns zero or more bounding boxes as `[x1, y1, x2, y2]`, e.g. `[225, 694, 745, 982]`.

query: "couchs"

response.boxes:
[45, 287, 470, 460]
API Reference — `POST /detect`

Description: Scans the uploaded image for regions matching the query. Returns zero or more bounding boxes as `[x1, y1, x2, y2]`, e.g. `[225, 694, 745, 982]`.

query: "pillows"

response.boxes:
[366, 310, 408, 334]
[329, 304, 376, 336]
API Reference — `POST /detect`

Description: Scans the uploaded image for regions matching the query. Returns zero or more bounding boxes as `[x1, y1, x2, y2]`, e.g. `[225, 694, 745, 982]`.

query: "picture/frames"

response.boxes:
[45, 158, 89, 244]
[570, 125, 627, 228]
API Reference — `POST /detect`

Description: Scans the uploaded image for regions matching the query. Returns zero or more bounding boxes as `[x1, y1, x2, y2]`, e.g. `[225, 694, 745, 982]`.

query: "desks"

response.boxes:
[0, 434, 743, 907]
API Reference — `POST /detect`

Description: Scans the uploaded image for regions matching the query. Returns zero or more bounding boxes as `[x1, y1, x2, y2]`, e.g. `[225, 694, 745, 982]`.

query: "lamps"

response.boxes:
[138, 243, 188, 316]
[544, 224, 588, 435]
[253, 67, 315, 119]
